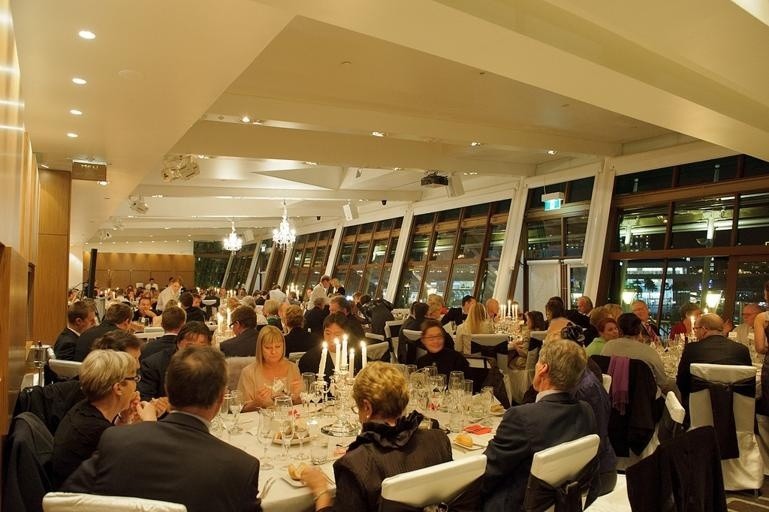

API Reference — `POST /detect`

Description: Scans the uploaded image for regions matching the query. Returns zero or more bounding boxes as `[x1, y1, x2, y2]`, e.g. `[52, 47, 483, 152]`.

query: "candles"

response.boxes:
[515, 304, 517, 318]
[288, 286, 290, 294]
[512, 304, 514, 318]
[317, 342, 328, 380]
[234, 290, 236, 298]
[503, 305, 506, 321]
[297, 290, 299, 299]
[291, 282, 294, 290]
[228, 311, 230, 328]
[508, 299, 510, 316]
[361, 341, 367, 368]
[220, 316, 222, 332]
[230, 290, 233, 298]
[296, 285, 298, 293]
[501, 304, 503, 319]
[335, 338, 341, 370]
[342, 334, 348, 368]
[218, 313, 220, 331]
[227, 291, 229, 298]
[227, 308, 229, 326]
[349, 348, 354, 378]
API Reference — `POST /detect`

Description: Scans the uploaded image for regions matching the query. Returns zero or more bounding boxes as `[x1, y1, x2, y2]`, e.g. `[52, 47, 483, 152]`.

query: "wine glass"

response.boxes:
[230, 390, 244, 435]
[400, 364, 494, 432]
[274, 397, 294, 422]
[259, 410, 275, 470]
[310, 383, 324, 416]
[295, 413, 310, 460]
[215, 397, 229, 436]
[279, 419, 295, 461]
[273, 377, 290, 401]
[302, 372, 315, 417]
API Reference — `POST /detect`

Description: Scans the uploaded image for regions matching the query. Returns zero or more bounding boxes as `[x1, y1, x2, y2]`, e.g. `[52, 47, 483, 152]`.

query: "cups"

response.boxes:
[310, 437, 329, 464]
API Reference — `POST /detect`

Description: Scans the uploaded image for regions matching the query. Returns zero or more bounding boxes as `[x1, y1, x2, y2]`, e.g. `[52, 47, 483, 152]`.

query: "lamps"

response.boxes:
[223, 221, 243, 256]
[272, 204, 295, 252]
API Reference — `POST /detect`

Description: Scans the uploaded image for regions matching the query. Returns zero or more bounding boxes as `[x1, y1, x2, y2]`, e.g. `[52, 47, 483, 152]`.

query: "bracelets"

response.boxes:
[312, 488, 331, 502]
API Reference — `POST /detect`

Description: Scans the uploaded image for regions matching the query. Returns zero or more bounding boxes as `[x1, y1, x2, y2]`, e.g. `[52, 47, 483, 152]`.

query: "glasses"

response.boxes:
[422, 334, 444, 341]
[692, 326, 702, 331]
[742, 312, 753, 317]
[228, 320, 237, 328]
[124, 374, 142, 383]
[350, 404, 361, 414]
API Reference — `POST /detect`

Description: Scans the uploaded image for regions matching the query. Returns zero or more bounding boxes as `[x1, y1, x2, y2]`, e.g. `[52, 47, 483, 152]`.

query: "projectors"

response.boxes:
[421, 175, 449, 189]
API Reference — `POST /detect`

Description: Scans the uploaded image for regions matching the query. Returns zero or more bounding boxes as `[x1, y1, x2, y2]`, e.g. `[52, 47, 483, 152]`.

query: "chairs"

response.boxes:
[381, 456, 486, 512]
[627, 426, 727, 512]
[690, 363, 769, 500]
[523, 434, 601, 511]
[48, 360, 83, 384]
[402, 329, 429, 365]
[366, 341, 389, 362]
[226, 357, 257, 389]
[42, 493, 186, 512]
[365, 333, 383, 345]
[611, 391, 687, 510]
[508, 331, 548, 403]
[470, 334, 508, 374]
[589, 355, 667, 472]
[289, 353, 305, 361]
[463, 355, 512, 410]
[754, 364, 769, 447]
[385, 321, 404, 363]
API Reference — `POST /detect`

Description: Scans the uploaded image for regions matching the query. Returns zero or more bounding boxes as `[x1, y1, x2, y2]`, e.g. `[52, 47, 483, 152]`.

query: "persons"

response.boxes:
[283, 271, 499, 403]
[300, 361, 453, 512]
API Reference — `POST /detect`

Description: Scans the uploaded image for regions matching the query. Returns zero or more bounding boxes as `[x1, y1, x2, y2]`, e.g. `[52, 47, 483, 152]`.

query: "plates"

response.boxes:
[269, 436, 313, 445]
[279, 470, 307, 488]
[449, 434, 494, 450]
